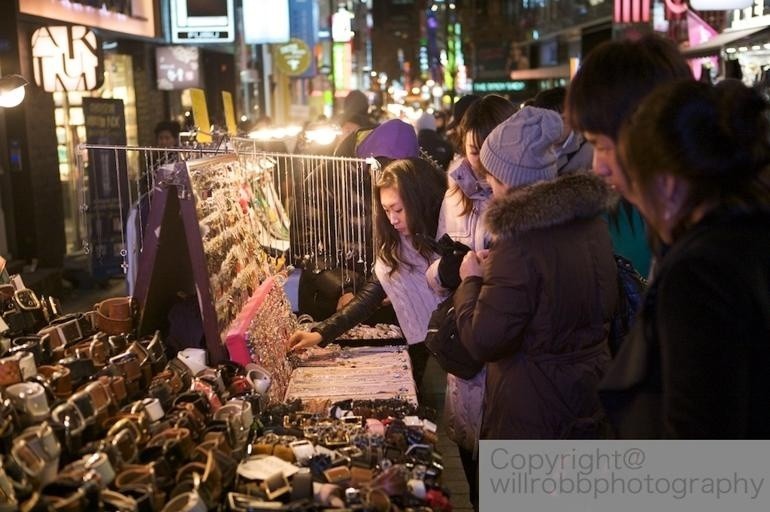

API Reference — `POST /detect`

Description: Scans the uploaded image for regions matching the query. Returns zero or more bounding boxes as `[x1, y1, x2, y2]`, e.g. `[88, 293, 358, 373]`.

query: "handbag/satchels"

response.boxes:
[612, 255, 648, 351]
[424, 281, 484, 380]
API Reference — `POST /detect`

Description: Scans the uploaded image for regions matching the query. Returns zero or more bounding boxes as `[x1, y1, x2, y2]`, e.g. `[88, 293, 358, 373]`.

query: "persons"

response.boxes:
[148, 32, 770, 511]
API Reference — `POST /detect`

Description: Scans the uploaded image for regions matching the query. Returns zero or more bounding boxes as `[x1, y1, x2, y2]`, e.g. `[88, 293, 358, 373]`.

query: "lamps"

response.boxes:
[0, 74, 29, 108]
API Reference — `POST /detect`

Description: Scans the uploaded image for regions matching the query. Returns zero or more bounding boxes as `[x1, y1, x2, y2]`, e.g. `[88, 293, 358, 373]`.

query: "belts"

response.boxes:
[0, 256, 453, 512]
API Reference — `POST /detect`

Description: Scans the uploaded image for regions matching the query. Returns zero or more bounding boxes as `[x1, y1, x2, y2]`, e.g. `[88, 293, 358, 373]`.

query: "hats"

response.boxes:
[446, 95, 482, 131]
[479, 105, 565, 187]
[418, 111, 439, 132]
